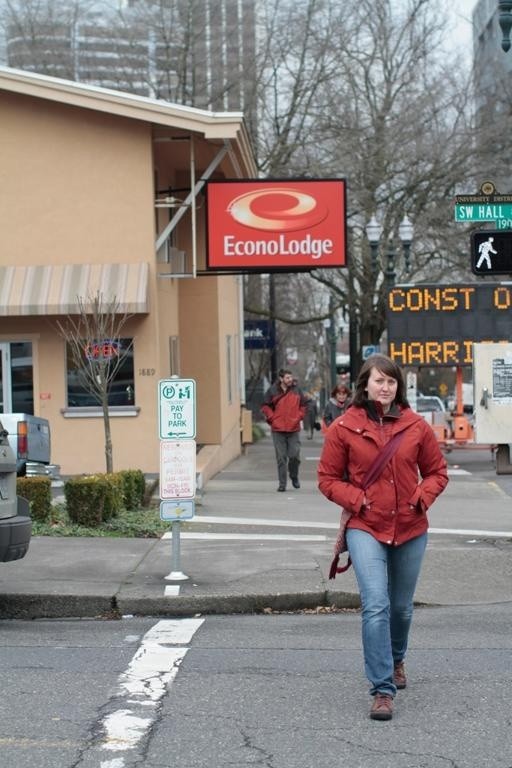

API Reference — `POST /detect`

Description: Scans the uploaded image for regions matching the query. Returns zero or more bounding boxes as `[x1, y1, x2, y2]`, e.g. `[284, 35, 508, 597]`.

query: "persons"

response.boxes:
[290, 379, 301, 395]
[260, 369, 306, 494]
[324, 384, 351, 427]
[317, 354, 447, 722]
[303, 392, 318, 440]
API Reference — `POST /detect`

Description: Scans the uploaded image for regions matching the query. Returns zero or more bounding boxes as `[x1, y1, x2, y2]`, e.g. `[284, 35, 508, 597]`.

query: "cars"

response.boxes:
[0, 418, 36, 568]
[418, 381, 475, 430]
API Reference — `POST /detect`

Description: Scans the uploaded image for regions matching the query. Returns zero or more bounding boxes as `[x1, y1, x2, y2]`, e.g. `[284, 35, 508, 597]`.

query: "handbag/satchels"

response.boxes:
[314, 422, 321, 431]
[264, 402, 276, 425]
[328, 506, 353, 580]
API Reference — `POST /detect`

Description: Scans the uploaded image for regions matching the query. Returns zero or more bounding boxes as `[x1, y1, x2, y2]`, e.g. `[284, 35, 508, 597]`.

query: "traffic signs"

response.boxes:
[455, 202, 512, 221]
[159, 379, 196, 438]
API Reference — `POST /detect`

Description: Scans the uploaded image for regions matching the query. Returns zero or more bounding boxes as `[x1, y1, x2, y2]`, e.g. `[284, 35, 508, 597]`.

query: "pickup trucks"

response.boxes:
[0, 413, 63, 484]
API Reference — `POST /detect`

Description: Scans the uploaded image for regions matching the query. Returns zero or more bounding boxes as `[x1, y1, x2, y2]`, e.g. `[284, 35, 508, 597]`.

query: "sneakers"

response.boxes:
[370, 692, 394, 721]
[277, 487, 285, 492]
[289, 474, 301, 488]
[392, 659, 407, 689]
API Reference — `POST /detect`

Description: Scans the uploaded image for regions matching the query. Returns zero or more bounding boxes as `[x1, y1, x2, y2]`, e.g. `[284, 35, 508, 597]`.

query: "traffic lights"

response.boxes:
[470, 229, 511, 276]
